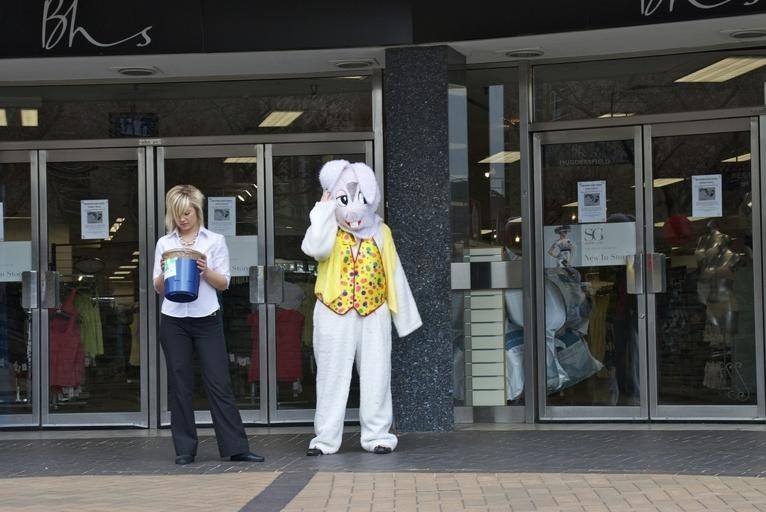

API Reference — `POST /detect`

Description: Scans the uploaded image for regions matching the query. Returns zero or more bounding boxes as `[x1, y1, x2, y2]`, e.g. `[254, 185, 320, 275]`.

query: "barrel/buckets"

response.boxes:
[161, 247, 203, 304]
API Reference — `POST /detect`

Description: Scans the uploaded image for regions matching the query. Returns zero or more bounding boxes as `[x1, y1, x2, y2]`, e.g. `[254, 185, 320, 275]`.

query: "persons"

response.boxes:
[154, 185, 266, 465]
[696, 217, 737, 333]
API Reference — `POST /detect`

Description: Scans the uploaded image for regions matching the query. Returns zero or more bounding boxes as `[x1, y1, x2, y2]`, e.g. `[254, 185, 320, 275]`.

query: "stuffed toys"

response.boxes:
[299, 160, 422, 453]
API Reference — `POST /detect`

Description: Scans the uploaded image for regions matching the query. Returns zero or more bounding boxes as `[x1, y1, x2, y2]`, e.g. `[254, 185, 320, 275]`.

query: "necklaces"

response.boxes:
[179, 232, 197, 246]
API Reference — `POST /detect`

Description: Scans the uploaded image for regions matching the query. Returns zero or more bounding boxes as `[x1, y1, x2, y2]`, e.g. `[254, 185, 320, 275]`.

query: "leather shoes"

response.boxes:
[175, 455, 193, 464]
[307, 449, 322, 455]
[374, 446, 391, 453]
[231, 451, 264, 461]
